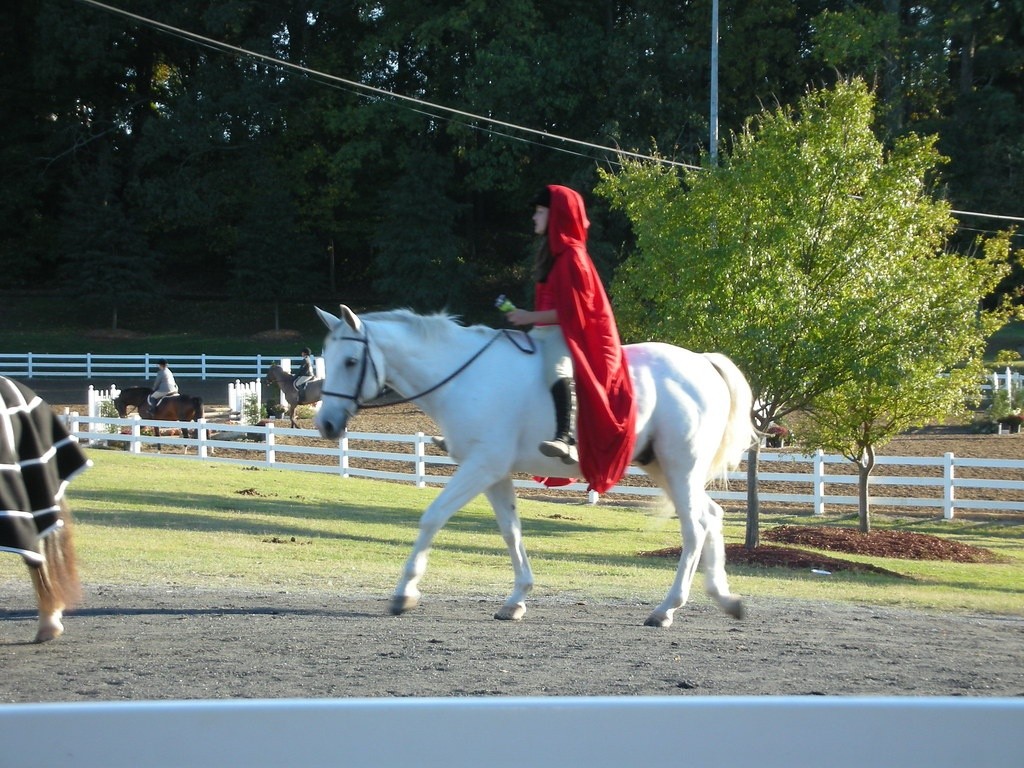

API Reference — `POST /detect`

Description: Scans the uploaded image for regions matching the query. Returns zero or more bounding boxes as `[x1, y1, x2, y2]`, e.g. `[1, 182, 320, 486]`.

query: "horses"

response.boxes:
[112, 387, 215, 457]
[1, 373, 83, 644]
[312, 304, 773, 628]
[260, 362, 325, 428]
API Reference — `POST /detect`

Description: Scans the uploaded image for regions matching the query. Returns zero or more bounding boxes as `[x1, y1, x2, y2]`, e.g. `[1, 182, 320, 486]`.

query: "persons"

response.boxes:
[506, 185, 590, 465]
[293, 347, 314, 402]
[146, 358, 178, 416]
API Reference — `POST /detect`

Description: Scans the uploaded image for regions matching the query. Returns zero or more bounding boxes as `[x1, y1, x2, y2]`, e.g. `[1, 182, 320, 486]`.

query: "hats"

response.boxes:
[527, 185, 552, 207]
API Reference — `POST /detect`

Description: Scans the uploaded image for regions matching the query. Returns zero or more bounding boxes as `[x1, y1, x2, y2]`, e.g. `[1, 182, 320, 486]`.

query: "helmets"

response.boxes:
[301, 346, 312, 356]
[158, 358, 169, 365]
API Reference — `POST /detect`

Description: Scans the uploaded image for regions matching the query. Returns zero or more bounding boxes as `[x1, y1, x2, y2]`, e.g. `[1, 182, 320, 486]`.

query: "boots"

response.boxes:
[149, 398, 159, 412]
[297, 390, 306, 402]
[538, 377, 580, 466]
